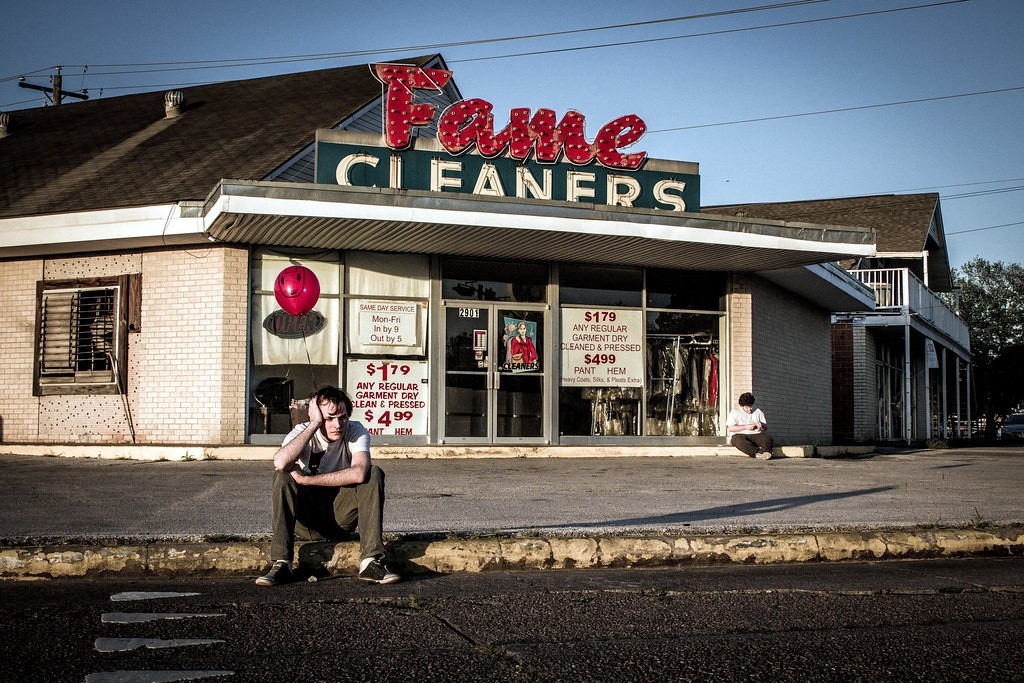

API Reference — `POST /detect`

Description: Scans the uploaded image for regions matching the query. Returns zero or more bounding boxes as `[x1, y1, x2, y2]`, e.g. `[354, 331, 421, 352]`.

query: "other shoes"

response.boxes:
[755, 451, 772, 460]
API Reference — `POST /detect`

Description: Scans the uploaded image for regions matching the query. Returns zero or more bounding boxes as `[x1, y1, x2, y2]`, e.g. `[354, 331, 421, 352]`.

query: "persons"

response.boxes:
[255, 384, 401, 586]
[724, 392, 774, 459]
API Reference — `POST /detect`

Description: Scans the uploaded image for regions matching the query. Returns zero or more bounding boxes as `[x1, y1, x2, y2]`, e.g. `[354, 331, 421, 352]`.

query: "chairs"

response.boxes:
[254, 380, 295, 434]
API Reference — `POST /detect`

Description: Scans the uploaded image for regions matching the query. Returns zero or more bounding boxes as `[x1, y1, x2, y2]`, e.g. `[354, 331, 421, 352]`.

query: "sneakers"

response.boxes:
[255, 561, 296, 586]
[358, 559, 401, 584]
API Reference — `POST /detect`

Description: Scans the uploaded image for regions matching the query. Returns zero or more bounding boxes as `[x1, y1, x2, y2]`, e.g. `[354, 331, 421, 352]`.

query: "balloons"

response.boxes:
[273, 264, 320, 317]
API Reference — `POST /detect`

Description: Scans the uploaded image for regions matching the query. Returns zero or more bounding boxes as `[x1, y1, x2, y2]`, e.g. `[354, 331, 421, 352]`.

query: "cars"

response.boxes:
[997, 413, 1024, 443]
